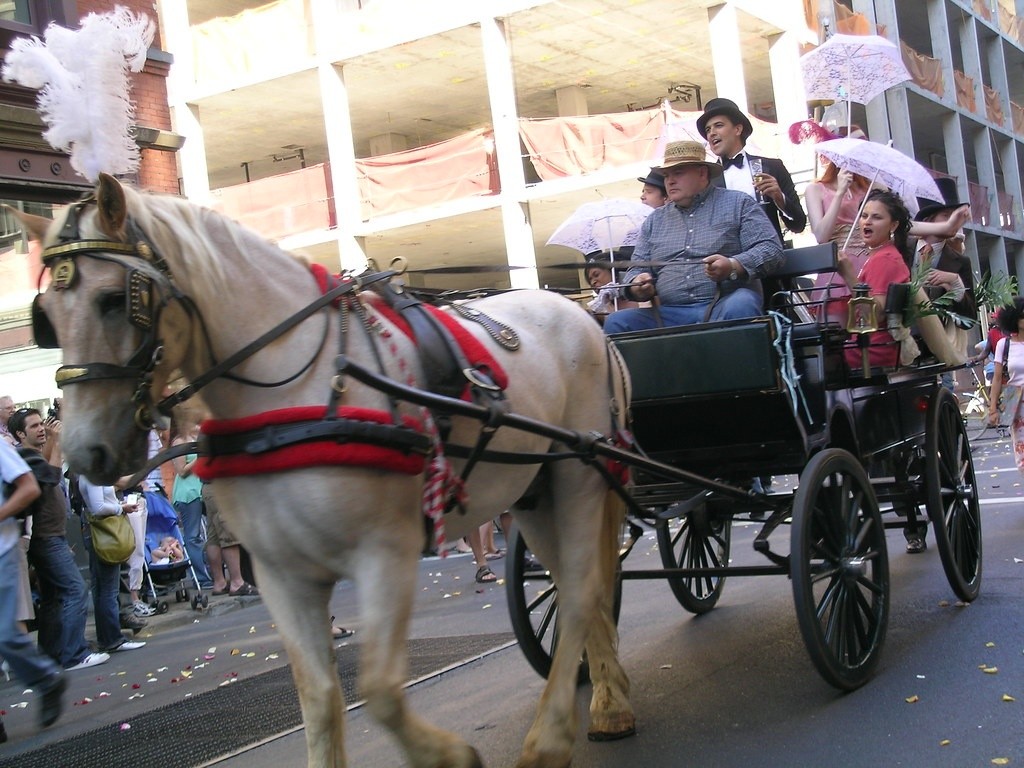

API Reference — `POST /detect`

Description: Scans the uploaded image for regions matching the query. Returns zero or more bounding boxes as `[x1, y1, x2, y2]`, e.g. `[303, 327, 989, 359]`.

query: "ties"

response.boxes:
[919, 243, 933, 265]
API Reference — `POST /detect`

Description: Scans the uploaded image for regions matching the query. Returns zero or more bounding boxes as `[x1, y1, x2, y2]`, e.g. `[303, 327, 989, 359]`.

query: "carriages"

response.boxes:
[0, 171, 987, 767]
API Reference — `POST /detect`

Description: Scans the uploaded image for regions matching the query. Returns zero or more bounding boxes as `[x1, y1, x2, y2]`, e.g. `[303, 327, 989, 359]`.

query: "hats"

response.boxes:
[993, 307, 1002, 317]
[655, 141, 724, 179]
[697, 98, 753, 140]
[914, 178, 970, 222]
[637, 166, 665, 188]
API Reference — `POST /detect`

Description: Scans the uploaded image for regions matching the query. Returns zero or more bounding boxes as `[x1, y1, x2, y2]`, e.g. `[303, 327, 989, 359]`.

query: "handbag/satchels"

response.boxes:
[89, 512, 137, 565]
[1002, 337, 1010, 384]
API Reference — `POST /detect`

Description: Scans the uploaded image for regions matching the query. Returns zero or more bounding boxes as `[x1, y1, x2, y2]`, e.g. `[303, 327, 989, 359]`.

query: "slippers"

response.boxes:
[212, 581, 230, 596]
[229, 580, 260, 596]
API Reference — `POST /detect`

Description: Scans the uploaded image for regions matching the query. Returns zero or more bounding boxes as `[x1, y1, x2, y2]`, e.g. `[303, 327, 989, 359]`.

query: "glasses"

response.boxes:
[1019, 312, 1024, 319]
[16, 409, 27, 429]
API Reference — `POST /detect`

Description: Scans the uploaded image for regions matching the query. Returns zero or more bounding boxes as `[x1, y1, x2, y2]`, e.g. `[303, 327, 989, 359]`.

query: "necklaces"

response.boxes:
[868, 238, 892, 250]
[856, 249, 877, 279]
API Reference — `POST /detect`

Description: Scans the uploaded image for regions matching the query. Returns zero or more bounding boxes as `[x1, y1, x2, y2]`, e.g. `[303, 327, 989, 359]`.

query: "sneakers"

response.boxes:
[64, 653, 110, 672]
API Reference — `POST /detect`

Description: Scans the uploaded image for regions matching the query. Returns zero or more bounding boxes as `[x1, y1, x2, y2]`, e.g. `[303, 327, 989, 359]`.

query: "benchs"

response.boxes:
[757, 238, 843, 340]
[841, 282, 971, 377]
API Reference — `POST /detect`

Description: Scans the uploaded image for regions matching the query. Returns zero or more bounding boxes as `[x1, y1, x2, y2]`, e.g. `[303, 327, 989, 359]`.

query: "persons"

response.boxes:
[0, 395, 355, 743]
[696, 98, 807, 301]
[953, 296, 1024, 477]
[584, 166, 668, 327]
[603, 141, 787, 335]
[804, 125, 977, 369]
[458, 510, 546, 583]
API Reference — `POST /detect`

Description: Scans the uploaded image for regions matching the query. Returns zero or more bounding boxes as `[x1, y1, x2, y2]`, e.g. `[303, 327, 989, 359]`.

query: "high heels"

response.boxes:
[1, 660, 15, 682]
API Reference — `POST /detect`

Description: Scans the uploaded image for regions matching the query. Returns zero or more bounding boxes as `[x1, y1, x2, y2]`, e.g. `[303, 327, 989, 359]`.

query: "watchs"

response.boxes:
[728, 258, 738, 281]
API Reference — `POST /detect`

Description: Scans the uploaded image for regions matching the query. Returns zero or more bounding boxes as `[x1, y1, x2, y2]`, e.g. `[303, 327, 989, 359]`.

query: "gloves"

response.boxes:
[587, 281, 619, 312]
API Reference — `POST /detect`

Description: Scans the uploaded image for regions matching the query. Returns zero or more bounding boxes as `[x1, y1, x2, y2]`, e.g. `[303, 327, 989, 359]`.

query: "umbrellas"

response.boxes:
[543, 197, 656, 311]
[811, 140, 945, 301]
[799, 33, 913, 138]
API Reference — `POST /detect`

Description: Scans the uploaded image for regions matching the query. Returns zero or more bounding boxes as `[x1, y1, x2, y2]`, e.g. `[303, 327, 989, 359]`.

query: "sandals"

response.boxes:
[524, 556, 544, 572]
[476, 565, 497, 583]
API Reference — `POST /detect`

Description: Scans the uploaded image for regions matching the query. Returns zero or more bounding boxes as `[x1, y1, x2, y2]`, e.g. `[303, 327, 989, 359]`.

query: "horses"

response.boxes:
[0, 172, 637, 768]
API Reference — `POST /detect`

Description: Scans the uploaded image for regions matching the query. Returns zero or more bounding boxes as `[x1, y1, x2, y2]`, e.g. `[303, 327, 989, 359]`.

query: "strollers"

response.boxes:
[122, 482, 208, 613]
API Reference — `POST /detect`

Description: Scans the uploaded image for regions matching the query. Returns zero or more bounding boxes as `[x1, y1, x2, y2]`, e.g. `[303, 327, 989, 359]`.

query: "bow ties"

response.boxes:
[721, 153, 744, 170]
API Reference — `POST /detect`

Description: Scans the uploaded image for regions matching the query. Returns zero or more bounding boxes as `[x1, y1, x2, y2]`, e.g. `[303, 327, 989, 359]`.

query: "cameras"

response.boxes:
[47, 403, 61, 423]
[127, 494, 138, 505]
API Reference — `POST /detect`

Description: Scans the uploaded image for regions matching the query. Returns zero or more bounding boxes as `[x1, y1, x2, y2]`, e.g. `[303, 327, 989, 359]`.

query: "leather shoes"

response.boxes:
[906, 538, 927, 553]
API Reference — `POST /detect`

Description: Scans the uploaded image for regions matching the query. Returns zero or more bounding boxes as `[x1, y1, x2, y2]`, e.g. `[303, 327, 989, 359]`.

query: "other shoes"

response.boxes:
[98, 641, 147, 653]
[131, 600, 156, 617]
[472, 549, 506, 565]
[119, 607, 148, 629]
[37, 671, 67, 725]
[456, 546, 473, 553]
